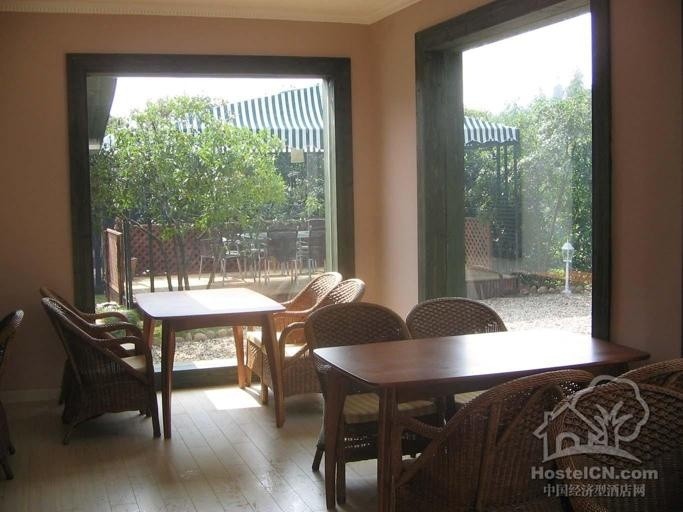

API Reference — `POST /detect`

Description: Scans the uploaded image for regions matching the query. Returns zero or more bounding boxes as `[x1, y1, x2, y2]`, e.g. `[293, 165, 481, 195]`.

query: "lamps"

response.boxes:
[561, 238, 575, 293]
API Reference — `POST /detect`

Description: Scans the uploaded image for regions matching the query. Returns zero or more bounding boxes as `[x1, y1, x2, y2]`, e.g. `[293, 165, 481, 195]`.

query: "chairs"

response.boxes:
[0, 270, 681, 512]
[190, 218, 324, 282]
